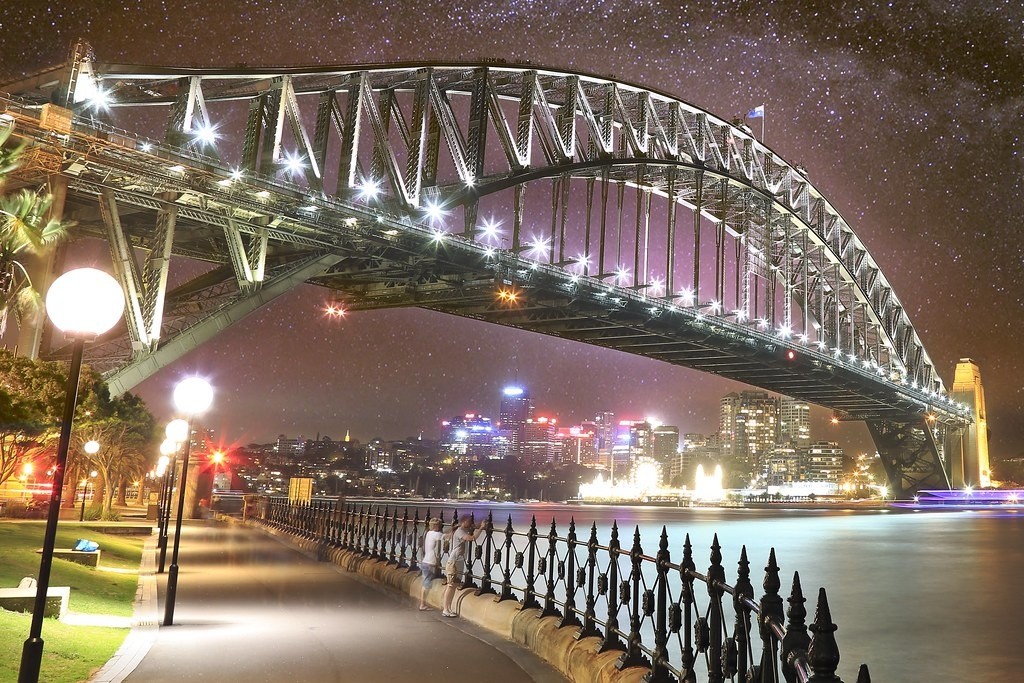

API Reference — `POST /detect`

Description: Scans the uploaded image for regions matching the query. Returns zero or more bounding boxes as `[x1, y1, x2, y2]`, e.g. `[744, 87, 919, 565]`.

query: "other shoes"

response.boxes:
[419, 605, 434, 611]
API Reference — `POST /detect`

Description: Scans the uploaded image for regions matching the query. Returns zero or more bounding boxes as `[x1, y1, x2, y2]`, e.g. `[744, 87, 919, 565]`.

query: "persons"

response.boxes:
[419, 517, 458, 611]
[442, 514, 486, 617]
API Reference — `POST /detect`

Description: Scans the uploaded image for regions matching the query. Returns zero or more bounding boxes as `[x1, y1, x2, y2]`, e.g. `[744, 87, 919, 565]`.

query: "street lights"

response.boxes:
[164, 374, 214, 625]
[21, 464, 34, 502]
[89, 472, 98, 499]
[156, 419, 190, 573]
[79, 441, 99, 522]
[15, 267, 128, 683]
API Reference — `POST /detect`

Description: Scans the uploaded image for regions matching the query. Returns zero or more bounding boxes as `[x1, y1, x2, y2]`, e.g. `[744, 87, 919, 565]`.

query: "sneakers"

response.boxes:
[442, 609, 457, 617]
